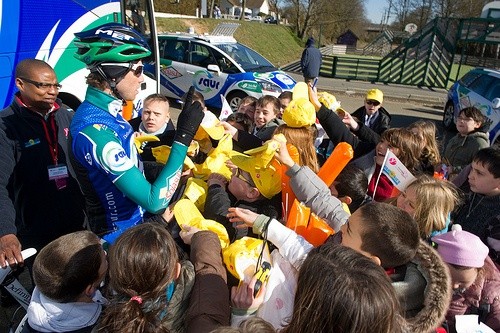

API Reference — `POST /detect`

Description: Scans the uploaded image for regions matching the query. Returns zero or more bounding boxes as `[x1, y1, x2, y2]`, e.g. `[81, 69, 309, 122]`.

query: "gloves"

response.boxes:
[172, 86, 206, 146]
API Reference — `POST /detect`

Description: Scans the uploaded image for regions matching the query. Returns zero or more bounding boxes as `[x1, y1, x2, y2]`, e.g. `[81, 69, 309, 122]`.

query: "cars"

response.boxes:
[441, 66, 500, 149]
[140, 30, 298, 113]
[229, 5, 277, 24]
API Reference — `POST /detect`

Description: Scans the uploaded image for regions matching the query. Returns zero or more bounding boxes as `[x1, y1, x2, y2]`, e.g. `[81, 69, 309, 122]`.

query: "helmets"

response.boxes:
[72, 22, 152, 65]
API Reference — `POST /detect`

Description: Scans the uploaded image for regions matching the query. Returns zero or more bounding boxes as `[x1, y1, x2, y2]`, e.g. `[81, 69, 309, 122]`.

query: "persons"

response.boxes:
[213, 4, 221, 19]
[131, 3, 148, 34]
[0, 22, 500, 333]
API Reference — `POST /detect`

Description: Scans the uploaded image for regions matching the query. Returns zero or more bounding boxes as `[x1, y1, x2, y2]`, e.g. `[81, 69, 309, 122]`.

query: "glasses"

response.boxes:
[96, 63, 144, 77]
[456, 117, 472, 122]
[19, 77, 62, 91]
[235, 167, 254, 188]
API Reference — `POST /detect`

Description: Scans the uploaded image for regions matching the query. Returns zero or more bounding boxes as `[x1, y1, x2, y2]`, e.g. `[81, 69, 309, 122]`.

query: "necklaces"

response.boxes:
[466, 193, 485, 216]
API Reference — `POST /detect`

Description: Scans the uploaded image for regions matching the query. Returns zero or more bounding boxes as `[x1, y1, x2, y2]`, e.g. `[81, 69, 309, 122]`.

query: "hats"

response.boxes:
[195, 111, 225, 140]
[307, 37, 315, 44]
[228, 153, 282, 199]
[133, 134, 159, 154]
[366, 88, 384, 104]
[223, 236, 271, 284]
[282, 82, 316, 128]
[431, 223, 489, 267]
[152, 134, 235, 211]
[244, 134, 287, 169]
[366, 100, 380, 106]
[171, 199, 229, 254]
